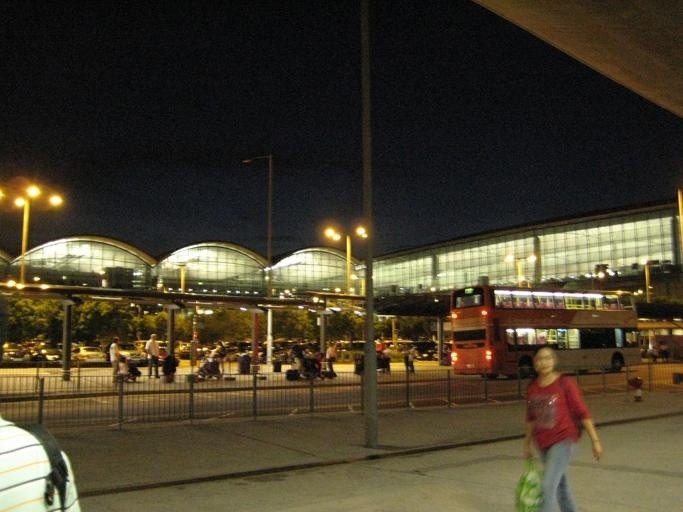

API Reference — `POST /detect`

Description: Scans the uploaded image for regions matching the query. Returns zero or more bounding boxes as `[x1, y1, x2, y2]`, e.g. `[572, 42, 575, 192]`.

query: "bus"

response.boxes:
[448, 284, 642, 380]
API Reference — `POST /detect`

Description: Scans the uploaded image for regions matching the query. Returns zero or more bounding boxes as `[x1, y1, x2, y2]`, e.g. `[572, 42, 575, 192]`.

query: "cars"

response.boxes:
[336, 338, 366, 361]
[120, 339, 209, 366]
[225, 338, 323, 363]
[1, 340, 107, 365]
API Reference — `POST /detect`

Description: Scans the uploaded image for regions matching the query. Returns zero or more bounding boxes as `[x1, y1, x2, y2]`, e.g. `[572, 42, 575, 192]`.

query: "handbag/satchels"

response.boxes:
[287, 370, 300, 380]
[516, 457, 544, 511]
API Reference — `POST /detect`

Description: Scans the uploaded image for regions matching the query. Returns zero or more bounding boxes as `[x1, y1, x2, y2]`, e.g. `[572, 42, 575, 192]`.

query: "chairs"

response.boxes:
[495, 302, 565, 309]
[532, 335, 544, 344]
[507, 337, 525, 345]
[603, 304, 624, 310]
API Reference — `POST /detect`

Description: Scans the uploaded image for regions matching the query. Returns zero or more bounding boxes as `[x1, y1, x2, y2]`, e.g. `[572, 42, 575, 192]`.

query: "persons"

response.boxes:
[282, 338, 338, 380]
[143, 333, 161, 379]
[214, 339, 225, 376]
[375, 342, 418, 374]
[523, 344, 603, 511]
[108, 337, 121, 384]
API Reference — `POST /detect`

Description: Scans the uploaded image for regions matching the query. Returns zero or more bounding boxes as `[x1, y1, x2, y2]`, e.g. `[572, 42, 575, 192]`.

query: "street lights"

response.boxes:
[242, 154, 273, 297]
[0, 176, 64, 285]
[506, 252, 538, 287]
[323, 222, 367, 291]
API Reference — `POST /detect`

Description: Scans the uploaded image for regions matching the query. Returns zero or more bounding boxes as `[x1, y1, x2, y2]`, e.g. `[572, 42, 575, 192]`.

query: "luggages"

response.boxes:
[239, 355, 251, 373]
[273, 360, 282, 372]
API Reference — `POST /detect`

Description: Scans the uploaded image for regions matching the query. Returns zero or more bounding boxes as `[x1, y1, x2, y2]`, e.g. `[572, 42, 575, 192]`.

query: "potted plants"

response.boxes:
[161, 356, 176, 383]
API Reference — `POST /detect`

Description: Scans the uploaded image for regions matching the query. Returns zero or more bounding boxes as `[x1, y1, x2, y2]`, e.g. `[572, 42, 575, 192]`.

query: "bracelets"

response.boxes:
[590, 439, 598, 443]
